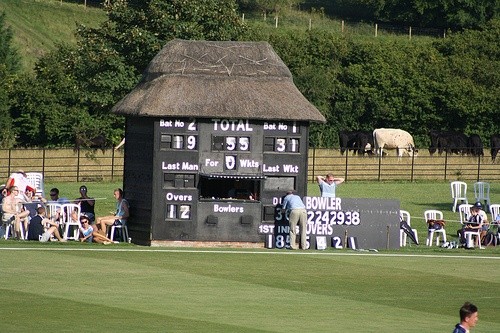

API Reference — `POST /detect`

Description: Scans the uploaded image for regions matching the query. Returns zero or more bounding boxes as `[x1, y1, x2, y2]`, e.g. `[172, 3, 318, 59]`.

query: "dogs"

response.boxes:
[426, 219, 446, 230]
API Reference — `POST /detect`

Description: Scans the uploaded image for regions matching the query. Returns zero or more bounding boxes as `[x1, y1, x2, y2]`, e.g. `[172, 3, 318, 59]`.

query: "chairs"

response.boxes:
[424, 210, 446, 246]
[451, 179, 500, 249]
[399, 210, 419, 246]
[0, 172, 129, 244]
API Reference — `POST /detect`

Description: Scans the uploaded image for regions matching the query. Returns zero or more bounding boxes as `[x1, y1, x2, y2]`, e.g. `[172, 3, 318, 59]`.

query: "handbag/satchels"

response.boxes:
[427, 219, 446, 229]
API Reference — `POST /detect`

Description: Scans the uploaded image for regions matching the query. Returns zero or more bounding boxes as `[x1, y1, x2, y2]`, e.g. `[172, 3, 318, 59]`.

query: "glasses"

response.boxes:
[81, 221, 87, 224]
[50, 194, 56, 196]
[81, 189, 86, 191]
[14, 190, 18, 192]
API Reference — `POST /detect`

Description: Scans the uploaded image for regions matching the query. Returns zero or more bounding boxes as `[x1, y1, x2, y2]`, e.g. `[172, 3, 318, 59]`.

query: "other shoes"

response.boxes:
[458, 243, 466, 248]
[7, 219, 11, 224]
[17, 236, 21, 240]
[287, 246, 296, 250]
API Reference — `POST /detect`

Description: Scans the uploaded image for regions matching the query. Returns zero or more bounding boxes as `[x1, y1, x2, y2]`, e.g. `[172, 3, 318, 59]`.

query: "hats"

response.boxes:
[17, 170, 24, 174]
[475, 202, 482, 209]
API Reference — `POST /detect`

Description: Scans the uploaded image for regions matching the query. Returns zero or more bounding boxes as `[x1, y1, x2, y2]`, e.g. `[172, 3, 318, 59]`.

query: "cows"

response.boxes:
[427, 130, 485, 162]
[372, 127, 419, 162]
[488, 132, 500, 162]
[337, 129, 375, 158]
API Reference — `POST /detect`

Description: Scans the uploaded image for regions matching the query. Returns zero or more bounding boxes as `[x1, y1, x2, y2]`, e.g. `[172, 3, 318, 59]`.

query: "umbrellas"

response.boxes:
[400, 216, 418, 245]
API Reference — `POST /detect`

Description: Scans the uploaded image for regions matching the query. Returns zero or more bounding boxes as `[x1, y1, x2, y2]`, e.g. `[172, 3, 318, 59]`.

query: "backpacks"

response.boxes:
[481, 231, 495, 246]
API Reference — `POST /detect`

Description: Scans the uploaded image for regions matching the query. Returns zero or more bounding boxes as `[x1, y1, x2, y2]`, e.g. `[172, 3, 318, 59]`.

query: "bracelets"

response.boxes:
[4, 187, 7, 190]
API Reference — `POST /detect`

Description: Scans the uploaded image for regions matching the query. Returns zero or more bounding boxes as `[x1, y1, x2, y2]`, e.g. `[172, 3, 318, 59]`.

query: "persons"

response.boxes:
[457, 201, 488, 248]
[2, 170, 41, 240]
[452, 302, 478, 333]
[97, 189, 130, 233]
[282, 191, 308, 250]
[27, 207, 65, 242]
[73, 185, 95, 222]
[316, 174, 344, 198]
[78, 216, 119, 245]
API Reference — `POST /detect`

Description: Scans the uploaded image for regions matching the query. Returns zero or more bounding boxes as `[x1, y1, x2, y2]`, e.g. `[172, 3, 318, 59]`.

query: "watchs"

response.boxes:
[47, 188, 67, 222]
